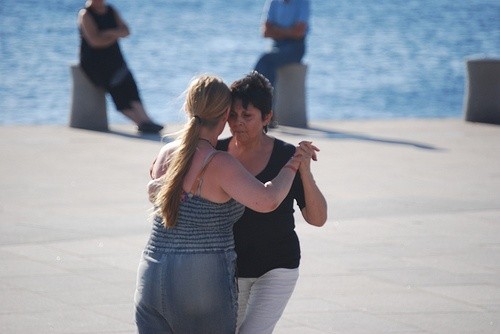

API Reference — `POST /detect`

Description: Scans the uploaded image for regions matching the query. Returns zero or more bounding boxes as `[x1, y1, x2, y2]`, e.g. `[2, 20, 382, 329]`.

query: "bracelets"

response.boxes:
[283, 164, 298, 172]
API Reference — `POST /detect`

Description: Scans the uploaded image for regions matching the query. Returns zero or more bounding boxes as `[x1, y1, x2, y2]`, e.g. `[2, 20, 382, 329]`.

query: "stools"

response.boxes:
[274, 62, 309, 130]
[66, 64, 109, 131]
[464, 56, 500, 123]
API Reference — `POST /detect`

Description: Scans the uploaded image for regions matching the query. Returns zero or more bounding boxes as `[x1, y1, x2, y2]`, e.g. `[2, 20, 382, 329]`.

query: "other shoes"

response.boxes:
[139, 122, 164, 132]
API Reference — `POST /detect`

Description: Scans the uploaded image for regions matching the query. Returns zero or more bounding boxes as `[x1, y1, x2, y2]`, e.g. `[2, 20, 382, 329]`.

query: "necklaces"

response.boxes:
[197, 136, 216, 149]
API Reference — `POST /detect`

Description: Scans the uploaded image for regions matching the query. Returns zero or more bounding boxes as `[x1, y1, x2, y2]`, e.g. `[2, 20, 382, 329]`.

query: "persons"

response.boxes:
[78, 0, 164, 133]
[214, 70, 327, 334]
[253, 0, 310, 86]
[132, 72, 320, 334]
